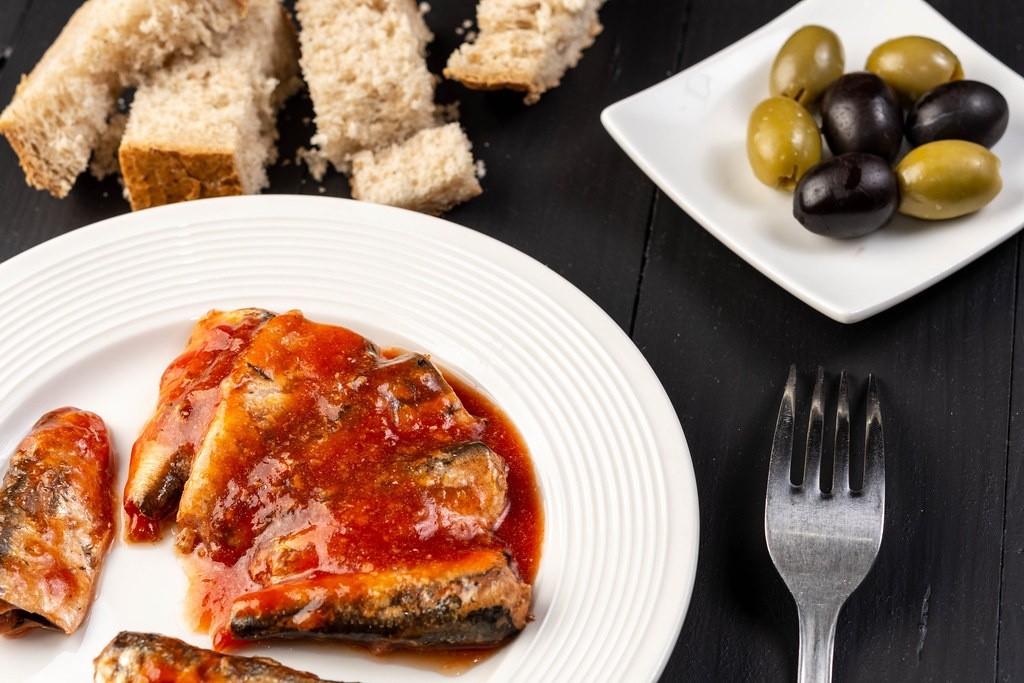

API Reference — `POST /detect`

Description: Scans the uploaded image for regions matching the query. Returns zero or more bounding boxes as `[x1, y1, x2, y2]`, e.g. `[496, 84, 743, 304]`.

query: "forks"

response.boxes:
[764, 363, 885, 683]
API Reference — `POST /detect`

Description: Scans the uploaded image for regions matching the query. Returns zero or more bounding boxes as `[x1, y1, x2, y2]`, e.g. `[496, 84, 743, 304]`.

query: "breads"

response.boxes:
[0, 0, 601, 208]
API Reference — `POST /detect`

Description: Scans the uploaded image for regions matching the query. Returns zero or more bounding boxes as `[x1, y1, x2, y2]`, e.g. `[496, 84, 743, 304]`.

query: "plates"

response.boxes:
[0, 195, 699, 683]
[600, 0, 1024, 324]
